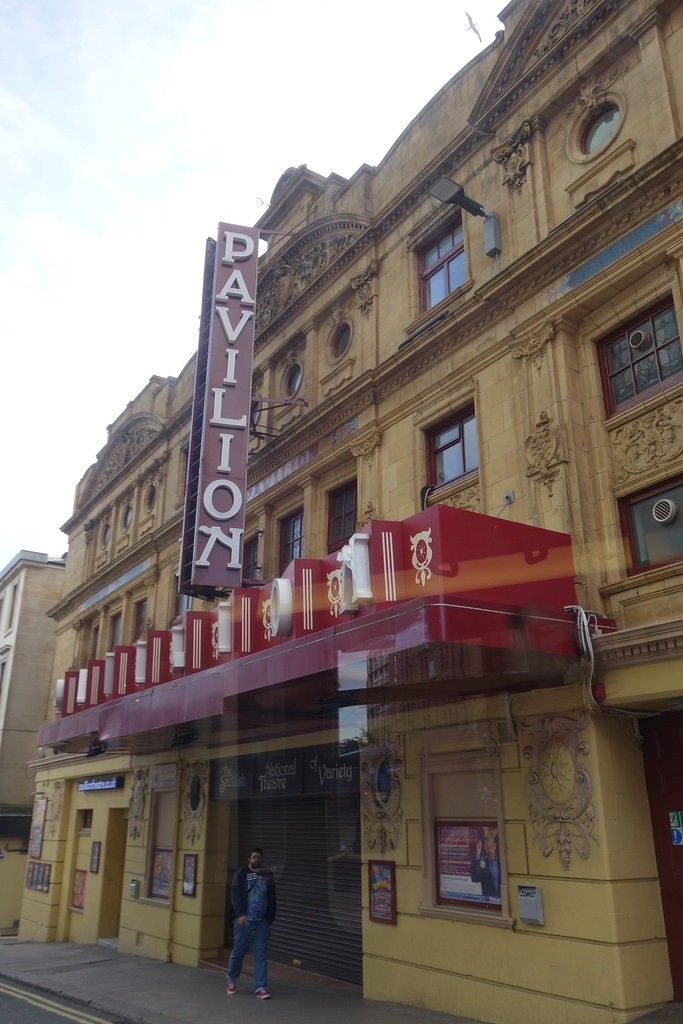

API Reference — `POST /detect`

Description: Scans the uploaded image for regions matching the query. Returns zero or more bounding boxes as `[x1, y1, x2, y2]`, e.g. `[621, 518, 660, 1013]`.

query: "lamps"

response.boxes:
[86, 742, 108, 758]
[429, 177, 487, 218]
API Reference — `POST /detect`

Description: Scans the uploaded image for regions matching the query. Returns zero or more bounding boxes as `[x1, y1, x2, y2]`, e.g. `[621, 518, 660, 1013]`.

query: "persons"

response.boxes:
[226, 846, 276, 1000]
[470, 830, 501, 899]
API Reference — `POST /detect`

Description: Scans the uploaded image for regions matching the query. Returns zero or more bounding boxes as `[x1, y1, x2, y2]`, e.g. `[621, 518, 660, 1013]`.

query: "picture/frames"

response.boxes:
[25, 797, 52, 893]
[182, 854, 198, 897]
[368, 859, 397, 925]
[90, 841, 101, 873]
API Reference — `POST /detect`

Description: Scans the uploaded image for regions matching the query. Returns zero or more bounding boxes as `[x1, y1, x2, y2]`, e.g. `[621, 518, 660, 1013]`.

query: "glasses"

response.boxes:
[250, 855, 264, 859]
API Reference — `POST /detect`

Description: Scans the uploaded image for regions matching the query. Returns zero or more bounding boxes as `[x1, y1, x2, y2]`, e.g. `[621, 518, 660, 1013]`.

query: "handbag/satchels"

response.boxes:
[229, 910, 236, 927]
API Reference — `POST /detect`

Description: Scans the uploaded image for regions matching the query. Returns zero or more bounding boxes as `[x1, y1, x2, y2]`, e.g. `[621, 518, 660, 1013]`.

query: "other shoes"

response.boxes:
[257, 990, 270, 1000]
[226, 981, 237, 994]
